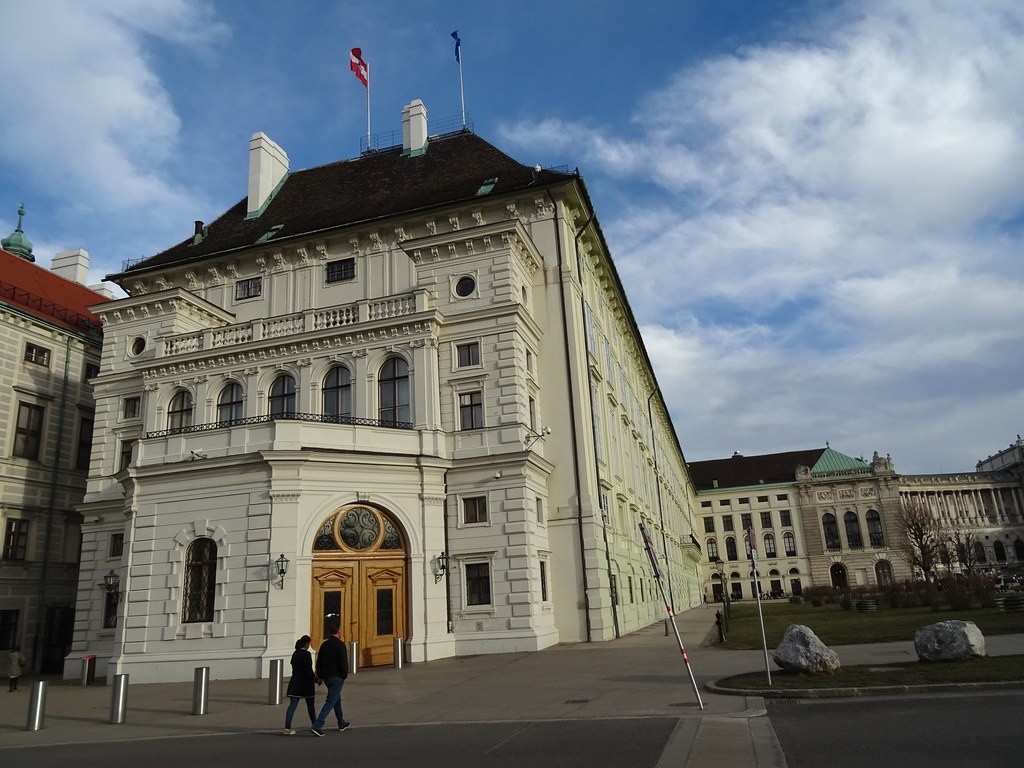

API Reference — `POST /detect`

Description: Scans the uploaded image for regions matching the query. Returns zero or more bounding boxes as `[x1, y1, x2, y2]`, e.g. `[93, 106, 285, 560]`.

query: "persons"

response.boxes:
[8, 646, 26, 692]
[994, 573, 1007, 594]
[310, 621, 351, 736]
[282, 635, 318, 734]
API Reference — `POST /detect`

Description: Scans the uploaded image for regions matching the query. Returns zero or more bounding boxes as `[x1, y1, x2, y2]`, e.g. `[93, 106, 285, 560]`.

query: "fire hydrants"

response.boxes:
[715, 610, 727, 642]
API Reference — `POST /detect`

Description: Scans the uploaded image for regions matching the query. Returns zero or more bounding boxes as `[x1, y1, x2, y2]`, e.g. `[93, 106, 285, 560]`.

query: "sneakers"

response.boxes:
[310, 727, 325, 737]
[338, 722, 351, 731]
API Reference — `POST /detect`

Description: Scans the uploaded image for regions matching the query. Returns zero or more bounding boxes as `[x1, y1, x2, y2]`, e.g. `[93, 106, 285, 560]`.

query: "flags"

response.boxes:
[451, 30, 461, 63]
[350, 47, 368, 86]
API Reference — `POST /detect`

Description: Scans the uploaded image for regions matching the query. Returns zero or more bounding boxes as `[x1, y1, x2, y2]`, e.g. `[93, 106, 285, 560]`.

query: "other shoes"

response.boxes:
[283, 728, 296, 734]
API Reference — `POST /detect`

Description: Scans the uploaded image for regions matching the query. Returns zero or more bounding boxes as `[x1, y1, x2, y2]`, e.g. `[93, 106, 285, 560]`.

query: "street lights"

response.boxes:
[715, 557, 728, 619]
